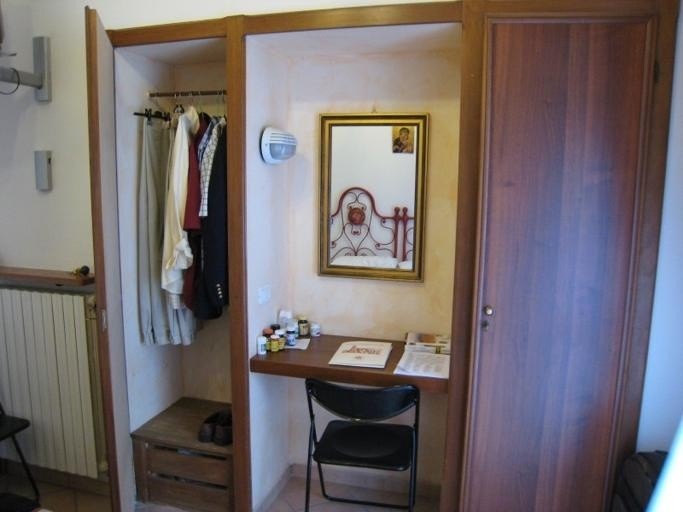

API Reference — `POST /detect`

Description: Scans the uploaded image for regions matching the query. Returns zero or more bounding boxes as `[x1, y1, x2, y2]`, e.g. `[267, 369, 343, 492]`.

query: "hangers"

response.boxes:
[133, 91, 225, 122]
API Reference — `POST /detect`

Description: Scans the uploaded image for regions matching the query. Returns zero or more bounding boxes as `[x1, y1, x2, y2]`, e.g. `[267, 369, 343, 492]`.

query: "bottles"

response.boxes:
[257, 316, 321, 356]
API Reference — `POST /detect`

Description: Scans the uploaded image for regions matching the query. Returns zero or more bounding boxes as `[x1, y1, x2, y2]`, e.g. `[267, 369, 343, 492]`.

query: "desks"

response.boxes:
[250, 335, 457, 394]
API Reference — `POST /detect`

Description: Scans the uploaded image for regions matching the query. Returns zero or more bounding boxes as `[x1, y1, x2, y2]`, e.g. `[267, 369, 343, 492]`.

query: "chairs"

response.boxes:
[306, 379, 419, 512]
[0, 404, 40, 503]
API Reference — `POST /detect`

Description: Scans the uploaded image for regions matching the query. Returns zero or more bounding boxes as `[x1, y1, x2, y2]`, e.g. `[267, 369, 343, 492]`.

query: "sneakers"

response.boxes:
[198, 412, 232, 446]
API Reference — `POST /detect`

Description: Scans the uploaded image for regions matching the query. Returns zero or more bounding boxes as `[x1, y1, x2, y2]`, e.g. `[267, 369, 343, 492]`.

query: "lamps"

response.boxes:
[261, 128, 298, 165]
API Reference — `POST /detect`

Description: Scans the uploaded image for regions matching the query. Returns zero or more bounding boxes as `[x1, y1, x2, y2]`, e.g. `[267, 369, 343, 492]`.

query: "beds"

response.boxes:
[329, 187, 415, 263]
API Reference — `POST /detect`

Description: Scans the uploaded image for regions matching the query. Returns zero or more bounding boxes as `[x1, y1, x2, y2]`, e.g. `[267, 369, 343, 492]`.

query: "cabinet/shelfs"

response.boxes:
[439, 0, 682, 512]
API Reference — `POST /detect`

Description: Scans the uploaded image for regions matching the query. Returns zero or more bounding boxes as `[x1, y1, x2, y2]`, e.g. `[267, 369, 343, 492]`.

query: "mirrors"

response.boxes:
[319, 111, 430, 283]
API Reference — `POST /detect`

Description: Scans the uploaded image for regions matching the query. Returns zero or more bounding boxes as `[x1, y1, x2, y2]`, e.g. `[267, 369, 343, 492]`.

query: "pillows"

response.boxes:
[330, 256, 397, 269]
[399, 260, 413, 269]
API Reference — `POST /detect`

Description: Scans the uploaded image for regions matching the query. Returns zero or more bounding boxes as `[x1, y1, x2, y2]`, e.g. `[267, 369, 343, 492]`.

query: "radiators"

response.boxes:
[0, 277, 109, 480]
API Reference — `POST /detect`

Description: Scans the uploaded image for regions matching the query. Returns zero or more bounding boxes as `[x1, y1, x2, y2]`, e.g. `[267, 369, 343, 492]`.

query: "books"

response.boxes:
[329, 340, 394, 368]
[393, 350, 450, 379]
